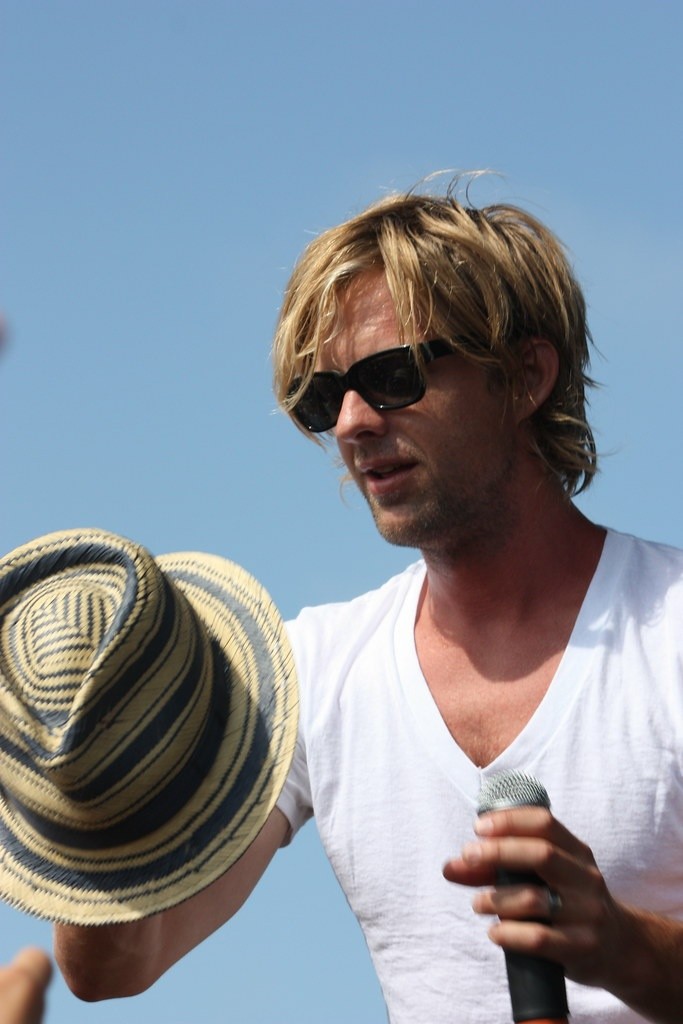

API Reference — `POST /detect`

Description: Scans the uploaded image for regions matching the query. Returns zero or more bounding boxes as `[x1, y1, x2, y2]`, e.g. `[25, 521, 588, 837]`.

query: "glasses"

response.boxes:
[286, 336, 486, 434]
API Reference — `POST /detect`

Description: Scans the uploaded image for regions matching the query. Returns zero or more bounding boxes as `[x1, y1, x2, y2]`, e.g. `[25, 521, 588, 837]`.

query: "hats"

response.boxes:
[0, 529, 301, 926]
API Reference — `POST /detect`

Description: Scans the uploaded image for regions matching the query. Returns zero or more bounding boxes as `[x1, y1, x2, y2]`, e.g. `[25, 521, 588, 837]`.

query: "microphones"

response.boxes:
[478, 772, 573, 1024]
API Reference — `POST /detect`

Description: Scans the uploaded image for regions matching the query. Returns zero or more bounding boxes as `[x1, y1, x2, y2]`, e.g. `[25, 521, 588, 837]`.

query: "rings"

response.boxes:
[548, 889, 562, 915]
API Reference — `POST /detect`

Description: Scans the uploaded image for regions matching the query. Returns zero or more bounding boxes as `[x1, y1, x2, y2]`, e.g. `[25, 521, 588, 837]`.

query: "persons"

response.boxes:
[48, 195, 683, 1023]
